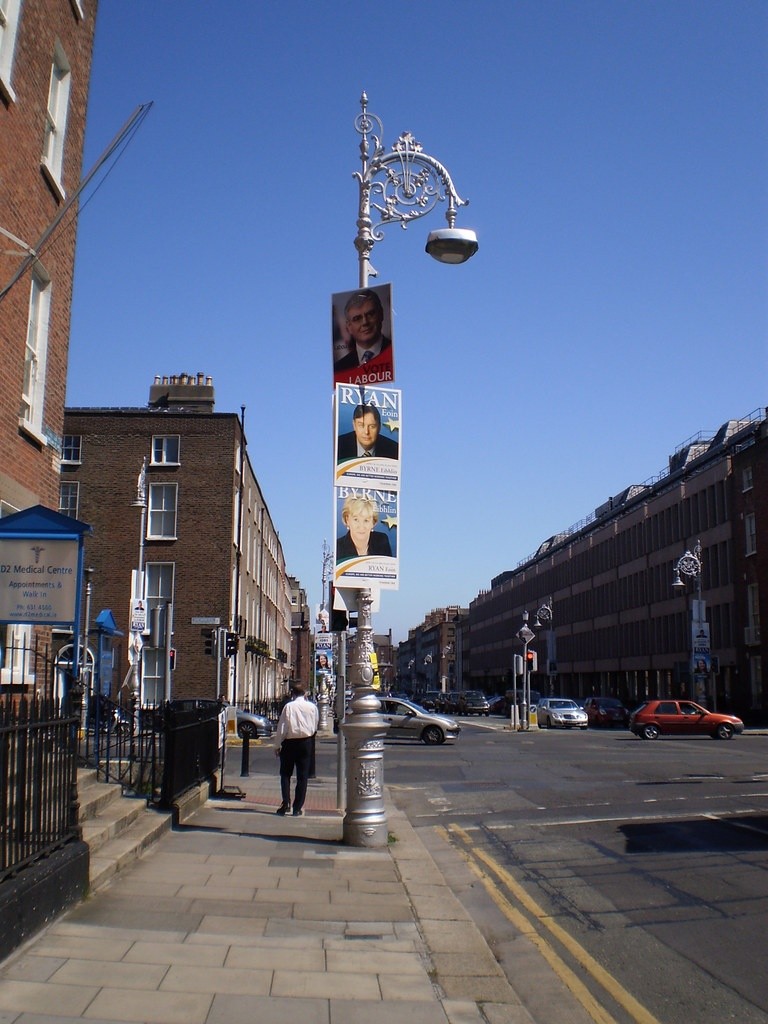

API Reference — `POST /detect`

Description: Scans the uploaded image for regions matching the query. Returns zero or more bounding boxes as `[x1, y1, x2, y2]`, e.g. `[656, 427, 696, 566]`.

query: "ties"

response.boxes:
[363, 451, 372, 457]
[364, 350, 374, 362]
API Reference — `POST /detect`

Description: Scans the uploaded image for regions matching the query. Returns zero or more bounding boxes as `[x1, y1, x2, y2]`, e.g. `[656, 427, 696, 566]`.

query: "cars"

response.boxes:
[582, 695, 630, 729]
[380, 683, 543, 718]
[167, 697, 275, 740]
[536, 697, 589, 731]
[345, 696, 462, 746]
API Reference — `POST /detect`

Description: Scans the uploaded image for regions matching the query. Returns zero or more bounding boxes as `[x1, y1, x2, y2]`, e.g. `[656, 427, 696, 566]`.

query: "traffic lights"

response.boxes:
[348, 609, 360, 629]
[204, 629, 217, 657]
[224, 630, 239, 658]
[527, 650, 538, 672]
[170, 648, 177, 671]
[328, 579, 348, 633]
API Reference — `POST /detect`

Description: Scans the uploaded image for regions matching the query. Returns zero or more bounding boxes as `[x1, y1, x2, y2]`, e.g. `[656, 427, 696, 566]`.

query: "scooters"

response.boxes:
[87, 692, 131, 739]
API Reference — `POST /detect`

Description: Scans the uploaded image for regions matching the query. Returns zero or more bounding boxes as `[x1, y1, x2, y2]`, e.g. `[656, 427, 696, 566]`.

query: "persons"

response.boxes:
[333, 288, 397, 558]
[692, 630, 710, 673]
[136, 600, 145, 609]
[318, 653, 330, 669]
[221, 684, 321, 816]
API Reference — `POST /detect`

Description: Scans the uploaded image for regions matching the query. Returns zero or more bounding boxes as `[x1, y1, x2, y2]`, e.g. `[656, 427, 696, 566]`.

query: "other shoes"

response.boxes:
[293, 808, 302, 815]
[277, 802, 290, 816]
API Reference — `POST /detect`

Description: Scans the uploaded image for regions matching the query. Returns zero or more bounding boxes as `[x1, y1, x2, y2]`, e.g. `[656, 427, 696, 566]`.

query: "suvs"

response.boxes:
[627, 698, 744, 741]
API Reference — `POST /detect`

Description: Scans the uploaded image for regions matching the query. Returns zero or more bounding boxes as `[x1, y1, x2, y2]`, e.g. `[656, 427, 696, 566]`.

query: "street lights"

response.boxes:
[334, 90, 482, 852]
[440, 641, 455, 691]
[534, 594, 557, 697]
[423, 649, 435, 690]
[75, 564, 97, 740]
[519, 609, 530, 733]
[408, 656, 417, 694]
[670, 537, 711, 711]
